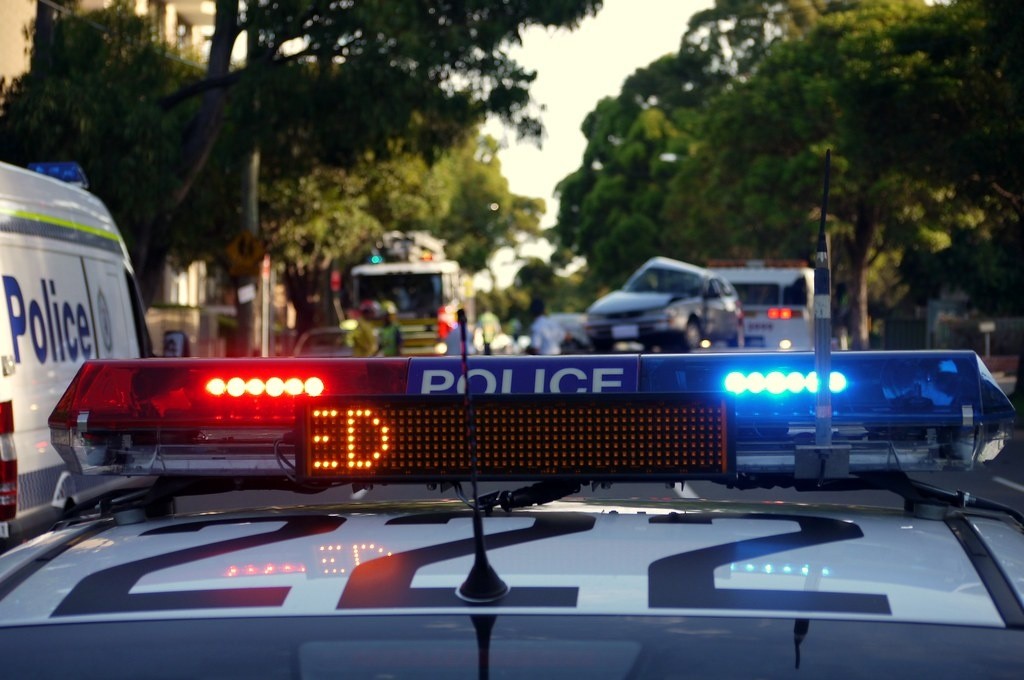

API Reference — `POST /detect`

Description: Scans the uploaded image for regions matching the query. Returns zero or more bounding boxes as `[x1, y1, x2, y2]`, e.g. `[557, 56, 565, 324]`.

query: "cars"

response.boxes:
[587, 255, 742, 354]
[544, 313, 595, 352]
[0, 347, 1022, 678]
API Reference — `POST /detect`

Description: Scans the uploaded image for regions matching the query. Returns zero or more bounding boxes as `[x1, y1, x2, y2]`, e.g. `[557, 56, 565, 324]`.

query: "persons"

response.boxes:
[351, 300, 403, 357]
[445, 311, 483, 355]
[526, 298, 566, 357]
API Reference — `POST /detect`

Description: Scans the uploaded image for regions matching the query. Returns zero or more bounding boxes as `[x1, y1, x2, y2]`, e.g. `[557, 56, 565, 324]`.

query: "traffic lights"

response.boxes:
[329, 270, 342, 292]
[370, 250, 384, 264]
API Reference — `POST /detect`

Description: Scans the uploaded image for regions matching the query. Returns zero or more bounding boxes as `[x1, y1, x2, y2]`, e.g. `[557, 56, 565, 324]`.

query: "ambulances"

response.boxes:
[704, 259, 818, 349]
[0, 152, 150, 554]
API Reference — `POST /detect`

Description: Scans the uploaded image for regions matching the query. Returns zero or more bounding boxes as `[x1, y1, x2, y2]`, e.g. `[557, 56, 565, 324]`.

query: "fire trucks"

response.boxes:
[349, 230, 465, 358]
